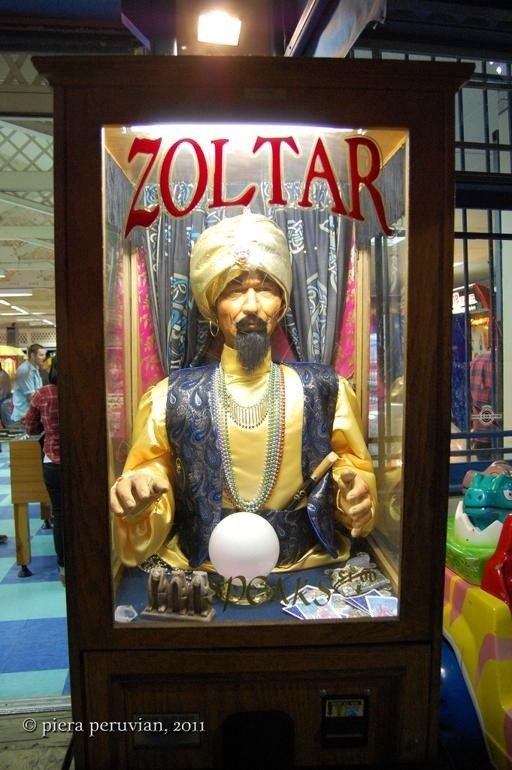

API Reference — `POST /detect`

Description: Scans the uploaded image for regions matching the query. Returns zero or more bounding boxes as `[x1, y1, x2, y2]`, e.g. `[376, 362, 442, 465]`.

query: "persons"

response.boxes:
[0, 343, 66, 588]
[110, 211, 379, 586]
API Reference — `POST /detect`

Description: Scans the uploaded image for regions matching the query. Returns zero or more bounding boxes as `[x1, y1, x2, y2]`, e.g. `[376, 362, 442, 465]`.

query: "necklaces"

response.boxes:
[212, 360, 286, 513]
